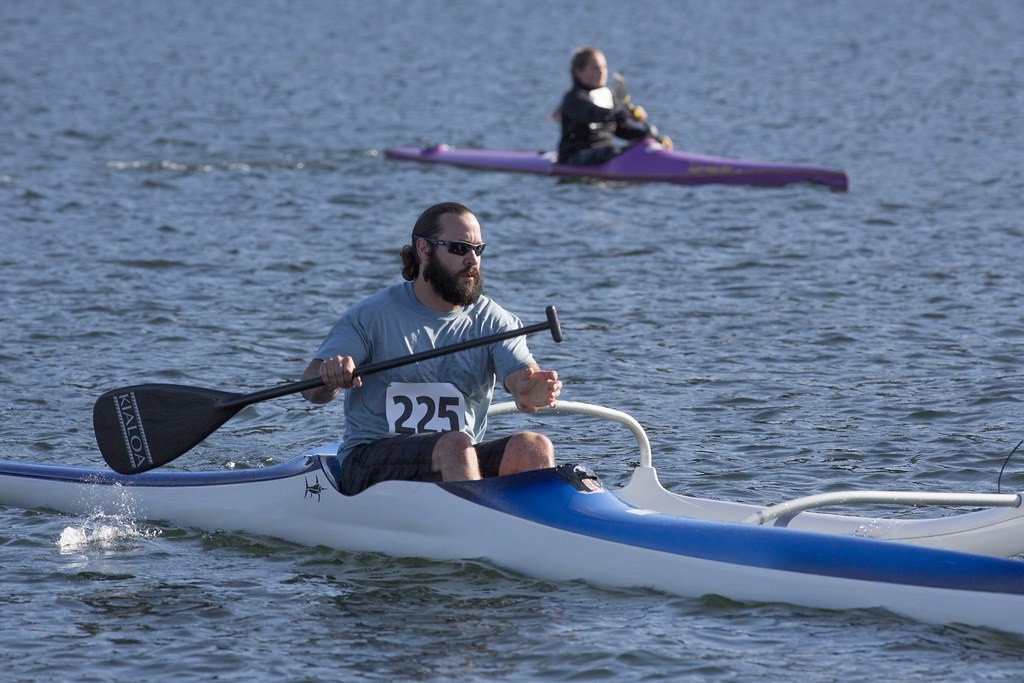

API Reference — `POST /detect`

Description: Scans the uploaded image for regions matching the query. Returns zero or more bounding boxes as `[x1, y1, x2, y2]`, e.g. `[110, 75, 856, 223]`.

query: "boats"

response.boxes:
[382, 136, 848, 193]
[0, 399, 1024, 633]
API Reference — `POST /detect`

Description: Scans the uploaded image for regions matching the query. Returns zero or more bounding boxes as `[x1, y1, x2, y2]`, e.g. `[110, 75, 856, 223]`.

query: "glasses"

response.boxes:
[414, 235, 486, 256]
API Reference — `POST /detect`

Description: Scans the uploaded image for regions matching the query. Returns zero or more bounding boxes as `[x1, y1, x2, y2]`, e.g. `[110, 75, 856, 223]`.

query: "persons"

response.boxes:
[301, 202, 562, 496]
[557, 48, 672, 166]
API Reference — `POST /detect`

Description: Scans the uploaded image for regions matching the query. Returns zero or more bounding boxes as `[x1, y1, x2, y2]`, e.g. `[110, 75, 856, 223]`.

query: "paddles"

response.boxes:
[611, 72, 674, 150]
[91, 303, 564, 474]
